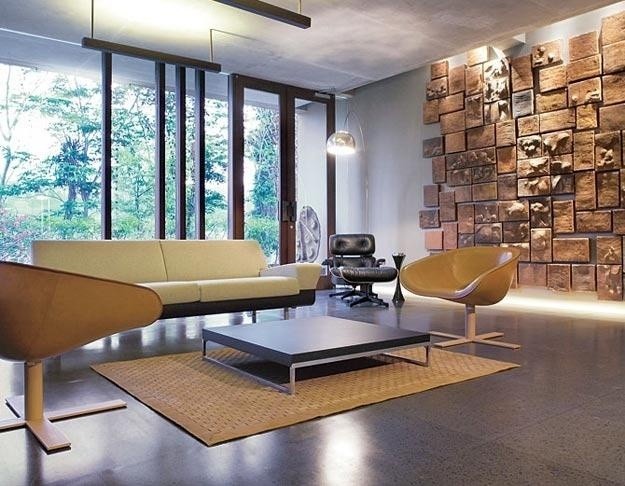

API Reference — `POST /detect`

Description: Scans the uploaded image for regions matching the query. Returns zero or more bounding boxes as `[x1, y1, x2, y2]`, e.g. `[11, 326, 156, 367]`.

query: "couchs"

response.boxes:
[31, 240, 322, 323]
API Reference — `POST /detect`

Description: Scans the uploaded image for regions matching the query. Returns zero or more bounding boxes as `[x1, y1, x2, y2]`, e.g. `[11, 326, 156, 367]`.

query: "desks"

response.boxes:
[202, 316, 431, 396]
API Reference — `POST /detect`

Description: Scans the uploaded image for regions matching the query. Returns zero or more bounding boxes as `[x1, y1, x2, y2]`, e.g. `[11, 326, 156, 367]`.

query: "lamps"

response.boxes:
[80, 0, 310, 74]
[326, 111, 370, 234]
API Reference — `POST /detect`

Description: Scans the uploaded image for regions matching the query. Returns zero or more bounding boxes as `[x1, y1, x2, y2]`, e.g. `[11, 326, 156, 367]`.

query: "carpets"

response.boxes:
[90, 346, 522, 447]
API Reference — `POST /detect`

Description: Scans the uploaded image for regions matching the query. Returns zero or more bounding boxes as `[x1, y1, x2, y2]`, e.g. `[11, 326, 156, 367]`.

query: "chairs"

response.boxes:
[400, 246, 522, 350]
[0, 259, 162, 451]
[328, 234, 398, 308]
